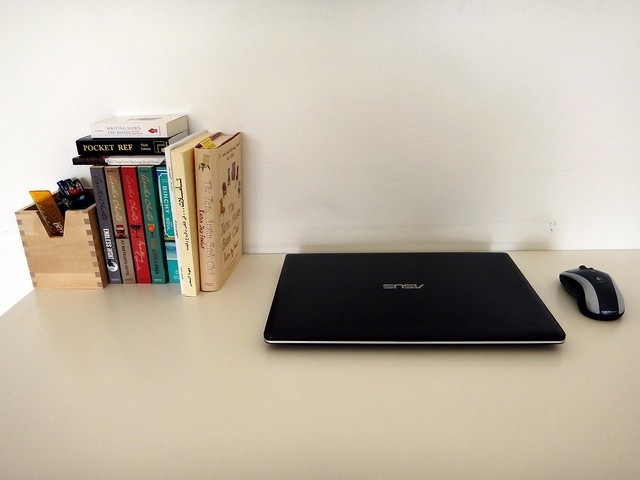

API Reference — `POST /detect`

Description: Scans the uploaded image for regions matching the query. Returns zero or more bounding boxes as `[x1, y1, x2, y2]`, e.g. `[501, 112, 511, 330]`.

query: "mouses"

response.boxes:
[559, 265, 625, 321]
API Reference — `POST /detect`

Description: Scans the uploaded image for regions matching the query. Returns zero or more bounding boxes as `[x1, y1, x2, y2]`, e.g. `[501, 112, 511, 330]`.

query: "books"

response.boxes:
[76, 130, 189, 155]
[89, 114, 189, 138]
[193, 131, 243, 291]
[104, 165, 137, 284]
[72, 155, 166, 166]
[136, 166, 169, 284]
[121, 166, 152, 284]
[164, 129, 208, 294]
[170, 130, 218, 297]
[156, 166, 180, 283]
[90, 165, 123, 284]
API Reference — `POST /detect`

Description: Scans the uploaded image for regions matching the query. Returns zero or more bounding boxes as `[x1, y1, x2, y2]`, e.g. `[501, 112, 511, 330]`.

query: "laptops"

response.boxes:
[264, 252, 565, 344]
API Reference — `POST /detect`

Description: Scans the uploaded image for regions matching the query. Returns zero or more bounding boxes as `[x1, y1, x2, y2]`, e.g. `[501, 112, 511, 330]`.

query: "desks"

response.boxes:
[0, 249, 640, 479]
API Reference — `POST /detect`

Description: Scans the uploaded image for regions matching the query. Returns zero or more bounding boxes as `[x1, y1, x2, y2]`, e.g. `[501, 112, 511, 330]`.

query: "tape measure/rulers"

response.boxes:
[28, 190, 65, 236]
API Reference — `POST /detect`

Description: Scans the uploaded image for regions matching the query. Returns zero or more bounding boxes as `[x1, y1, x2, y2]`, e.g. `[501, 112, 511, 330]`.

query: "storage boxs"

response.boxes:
[15, 187, 109, 290]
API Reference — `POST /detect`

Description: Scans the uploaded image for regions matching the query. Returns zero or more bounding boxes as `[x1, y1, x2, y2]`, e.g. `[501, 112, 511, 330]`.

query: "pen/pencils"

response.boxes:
[64, 178, 74, 190]
[73, 179, 84, 192]
[57, 180, 71, 198]
